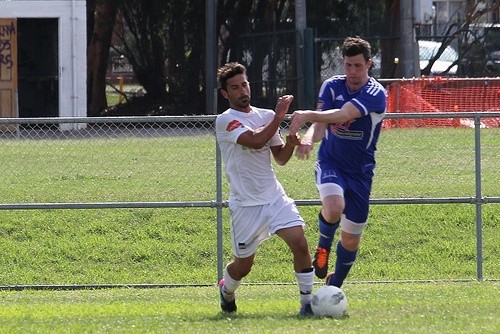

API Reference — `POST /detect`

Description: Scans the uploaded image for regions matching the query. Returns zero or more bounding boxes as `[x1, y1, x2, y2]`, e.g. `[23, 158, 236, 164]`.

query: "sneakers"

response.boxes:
[219, 278, 237, 313]
[326, 273, 340, 288]
[299, 302, 315, 318]
[313, 246, 330, 279]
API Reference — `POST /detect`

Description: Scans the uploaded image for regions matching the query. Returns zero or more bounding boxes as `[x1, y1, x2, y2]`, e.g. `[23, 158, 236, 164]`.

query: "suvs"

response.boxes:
[458, 25, 500, 77]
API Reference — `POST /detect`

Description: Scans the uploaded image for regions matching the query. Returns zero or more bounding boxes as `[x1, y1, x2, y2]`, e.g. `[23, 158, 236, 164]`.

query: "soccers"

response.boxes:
[310, 285, 348, 318]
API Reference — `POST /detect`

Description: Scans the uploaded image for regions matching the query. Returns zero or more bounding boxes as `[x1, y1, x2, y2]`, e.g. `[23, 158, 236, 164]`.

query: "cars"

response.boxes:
[371, 39, 458, 77]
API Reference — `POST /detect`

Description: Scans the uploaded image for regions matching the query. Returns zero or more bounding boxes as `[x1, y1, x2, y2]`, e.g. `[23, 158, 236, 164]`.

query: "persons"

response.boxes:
[285, 38, 388, 290]
[215, 62, 320, 316]
